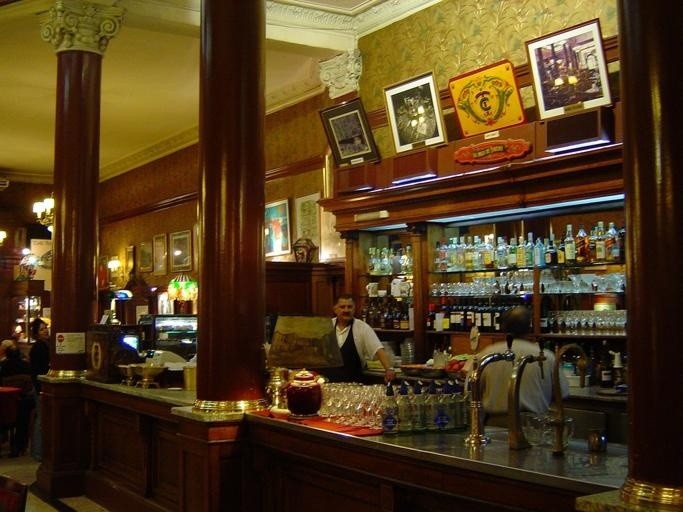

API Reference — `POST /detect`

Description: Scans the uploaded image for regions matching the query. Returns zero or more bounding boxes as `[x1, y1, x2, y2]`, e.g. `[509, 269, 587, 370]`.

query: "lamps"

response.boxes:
[105, 282, 134, 325]
[107, 254, 121, 274]
[19, 245, 41, 281]
[266, 313, 344, 419]
[32, 191, 54, 235]
[165, 270, 200, 314]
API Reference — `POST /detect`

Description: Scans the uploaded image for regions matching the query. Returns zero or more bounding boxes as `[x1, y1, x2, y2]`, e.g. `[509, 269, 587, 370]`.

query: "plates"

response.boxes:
[362, 354, 402, 366]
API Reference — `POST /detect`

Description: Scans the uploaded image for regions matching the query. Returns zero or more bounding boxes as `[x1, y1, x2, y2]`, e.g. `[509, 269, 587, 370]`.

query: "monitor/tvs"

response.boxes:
[124, 333, 139, 351]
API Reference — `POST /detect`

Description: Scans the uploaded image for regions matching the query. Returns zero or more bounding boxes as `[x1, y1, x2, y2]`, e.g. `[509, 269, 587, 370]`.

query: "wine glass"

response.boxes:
[116, 363, 169, 388]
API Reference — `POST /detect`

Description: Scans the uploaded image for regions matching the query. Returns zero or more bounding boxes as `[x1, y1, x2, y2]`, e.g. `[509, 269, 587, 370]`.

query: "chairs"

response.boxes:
[0, 474, 28, 512]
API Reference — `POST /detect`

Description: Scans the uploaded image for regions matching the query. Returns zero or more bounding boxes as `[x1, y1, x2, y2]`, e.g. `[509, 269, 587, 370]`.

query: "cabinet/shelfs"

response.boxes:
[346, 193, 627, 339]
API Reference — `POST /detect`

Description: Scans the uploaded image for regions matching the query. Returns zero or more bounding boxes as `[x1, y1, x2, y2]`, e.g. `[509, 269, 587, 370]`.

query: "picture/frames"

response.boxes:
[449, 58, 528, 139]
[121, 229, 194, 278]
[315, 95, 381, 167]
[524, 17, 617, 122]
[381, 68, 448, 158]
[262, 197, 290, 258]
[292, 191, 322, 260]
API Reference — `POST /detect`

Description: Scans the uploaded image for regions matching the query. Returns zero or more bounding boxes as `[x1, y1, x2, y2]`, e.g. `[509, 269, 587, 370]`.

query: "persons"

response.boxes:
[0, 339, 36, 456]
[29, 318, 50, 461]
[319, 294, 397, 385]
[465, 306, 569, 416]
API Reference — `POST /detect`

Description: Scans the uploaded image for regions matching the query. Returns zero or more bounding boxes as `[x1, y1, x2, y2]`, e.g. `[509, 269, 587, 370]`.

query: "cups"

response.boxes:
[400, 337, 416, 364]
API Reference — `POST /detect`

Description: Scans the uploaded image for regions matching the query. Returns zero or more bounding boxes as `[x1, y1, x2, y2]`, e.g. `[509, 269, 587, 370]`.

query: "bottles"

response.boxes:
[24, 296, 39, 311]
[266, 367, 479, 437]
[431, 342, 452, 358]
[184, 366, 197, 391]
[561, 340, 628, 390]
[361, 222, 627, 335]
[586, 428, 609, 451]
[520, 411, 574, 450]
